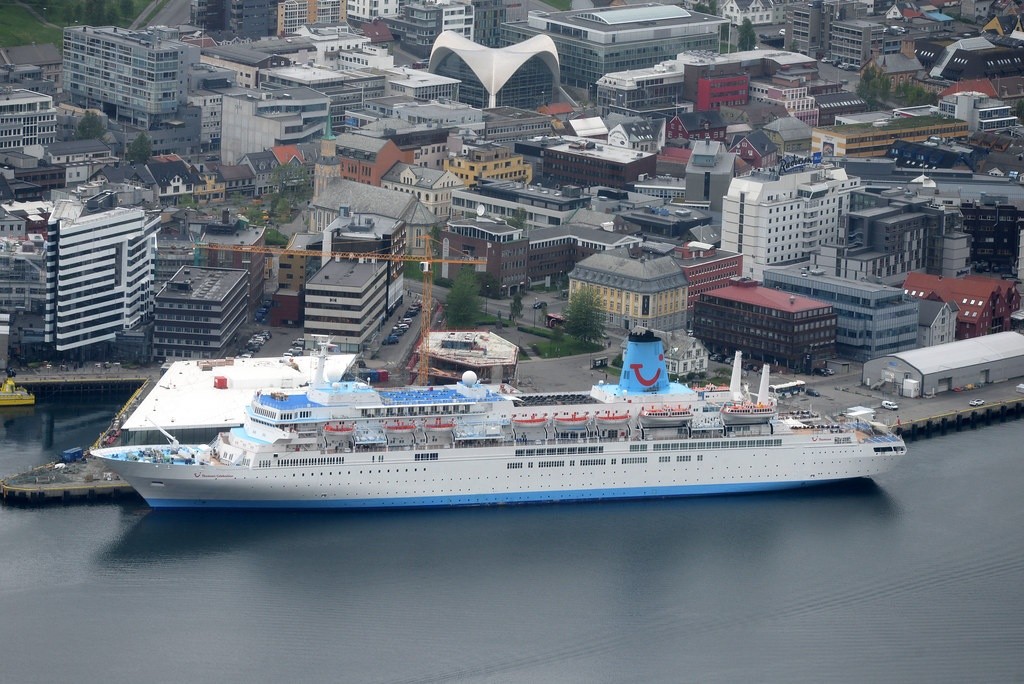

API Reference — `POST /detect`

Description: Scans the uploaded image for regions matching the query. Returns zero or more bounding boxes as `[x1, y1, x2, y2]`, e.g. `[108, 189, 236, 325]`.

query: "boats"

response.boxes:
[555, 414, 589, 424]
[424, 420, 455, 431]
[512, 415, 548, 428]
[720, 401, 774, 424]
[383, 421, 415, 432]
[598, 413, 631, 425]
[638, 408, 694, 428]
[320, 424, 354, 435]
[87, 329, 908, 509]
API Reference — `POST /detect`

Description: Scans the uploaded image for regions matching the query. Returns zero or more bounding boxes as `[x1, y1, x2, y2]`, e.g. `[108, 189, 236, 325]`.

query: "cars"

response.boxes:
[882, 401, 899, 410]
[238, 298, 305, 359]
[731, 22, 785, 50]
[260, 210, 270, 221]
[812, 368, 827, 376]
[806, 389, 819, 396]
[204, 155, 216, 161]
[533, 301, 547, 309]
[381, 298, 423, 345]
[821, 57, 860, 70]
[708, 351, 772, 374]
[826, 369, 836, 375]
[688, 331, 693, 336]
[969, 399, 984, 406]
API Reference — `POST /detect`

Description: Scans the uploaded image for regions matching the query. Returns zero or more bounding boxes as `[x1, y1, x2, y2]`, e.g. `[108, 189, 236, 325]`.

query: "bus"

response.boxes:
[768, 380, 805, 400]
[545, 312, 565, 328]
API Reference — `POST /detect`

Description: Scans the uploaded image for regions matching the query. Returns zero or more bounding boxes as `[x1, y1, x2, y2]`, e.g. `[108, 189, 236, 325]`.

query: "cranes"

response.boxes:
[189, 234, 488, 386]
